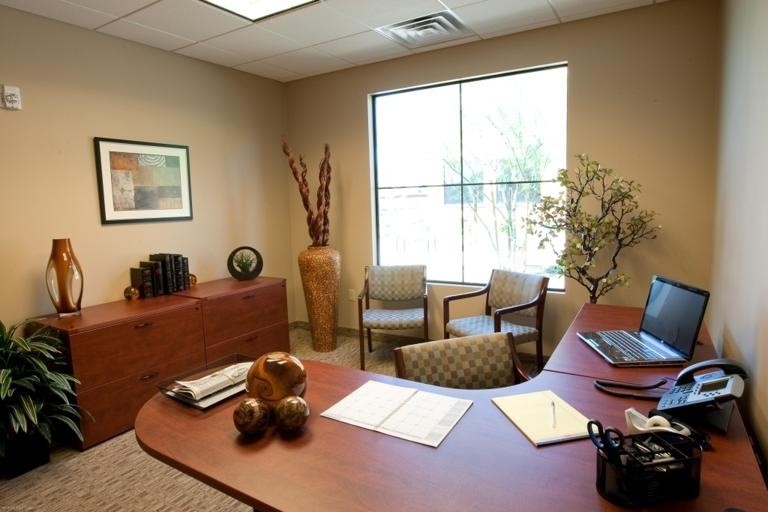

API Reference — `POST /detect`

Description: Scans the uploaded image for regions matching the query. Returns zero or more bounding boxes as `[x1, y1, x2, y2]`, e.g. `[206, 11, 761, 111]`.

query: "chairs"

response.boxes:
[392, 331, 533, 390]
[442, 267, 550, 375]
[357, 265, 429, 371]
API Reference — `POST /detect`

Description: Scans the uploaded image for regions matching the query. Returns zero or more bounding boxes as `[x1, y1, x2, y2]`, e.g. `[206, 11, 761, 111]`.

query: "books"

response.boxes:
[171, 254, 184, 292]
[148, 254, 173, 295]
[168, 254, 177, 294]
[130, 266, 154, 299]
[138, 261, 164, 297]
[182, 256, 190, 291]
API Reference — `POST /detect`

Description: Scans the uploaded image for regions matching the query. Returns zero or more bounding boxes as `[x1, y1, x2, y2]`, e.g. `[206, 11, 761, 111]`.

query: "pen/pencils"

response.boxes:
[552, 402, 556, 430]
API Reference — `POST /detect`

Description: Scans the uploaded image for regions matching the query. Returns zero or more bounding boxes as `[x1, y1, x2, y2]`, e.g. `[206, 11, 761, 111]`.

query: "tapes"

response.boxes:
[646, 416, 671, 428]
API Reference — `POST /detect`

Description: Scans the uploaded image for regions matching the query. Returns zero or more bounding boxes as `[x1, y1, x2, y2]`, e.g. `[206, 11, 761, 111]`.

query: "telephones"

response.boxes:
[593, 358, 751, 412]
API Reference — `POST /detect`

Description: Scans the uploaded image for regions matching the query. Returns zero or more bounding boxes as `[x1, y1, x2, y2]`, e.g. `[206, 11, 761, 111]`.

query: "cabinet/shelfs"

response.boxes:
[25, 277, 290, 451]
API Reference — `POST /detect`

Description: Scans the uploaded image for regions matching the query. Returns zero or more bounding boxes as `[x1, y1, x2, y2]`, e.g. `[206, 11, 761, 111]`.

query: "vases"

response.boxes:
[44, 236, 87, 318]
[298, 245, 342, 353]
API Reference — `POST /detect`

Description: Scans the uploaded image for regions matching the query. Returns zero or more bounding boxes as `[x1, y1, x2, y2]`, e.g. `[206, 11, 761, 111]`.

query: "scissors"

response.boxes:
[588, 419, 628, 495]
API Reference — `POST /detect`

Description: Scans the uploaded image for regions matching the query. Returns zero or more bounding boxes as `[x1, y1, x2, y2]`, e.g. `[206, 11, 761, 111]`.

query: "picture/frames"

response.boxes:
[94, 137, 193, 225]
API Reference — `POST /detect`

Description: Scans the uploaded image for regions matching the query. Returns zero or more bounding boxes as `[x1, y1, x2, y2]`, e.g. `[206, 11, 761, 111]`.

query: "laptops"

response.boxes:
[576, 274, 710, 367]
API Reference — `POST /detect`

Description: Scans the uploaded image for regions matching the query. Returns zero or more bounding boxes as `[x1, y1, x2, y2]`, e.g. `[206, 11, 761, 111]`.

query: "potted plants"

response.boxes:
[1, 318, 98, 481]
[228, 247, 263, 281]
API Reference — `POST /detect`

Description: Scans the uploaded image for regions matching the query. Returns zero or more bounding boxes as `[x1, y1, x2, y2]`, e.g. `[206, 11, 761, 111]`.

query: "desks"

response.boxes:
[134, 302, 768, 512]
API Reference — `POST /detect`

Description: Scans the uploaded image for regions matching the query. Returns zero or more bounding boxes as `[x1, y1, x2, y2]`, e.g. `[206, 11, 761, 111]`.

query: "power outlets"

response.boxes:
[347, 288, 356, 302]
